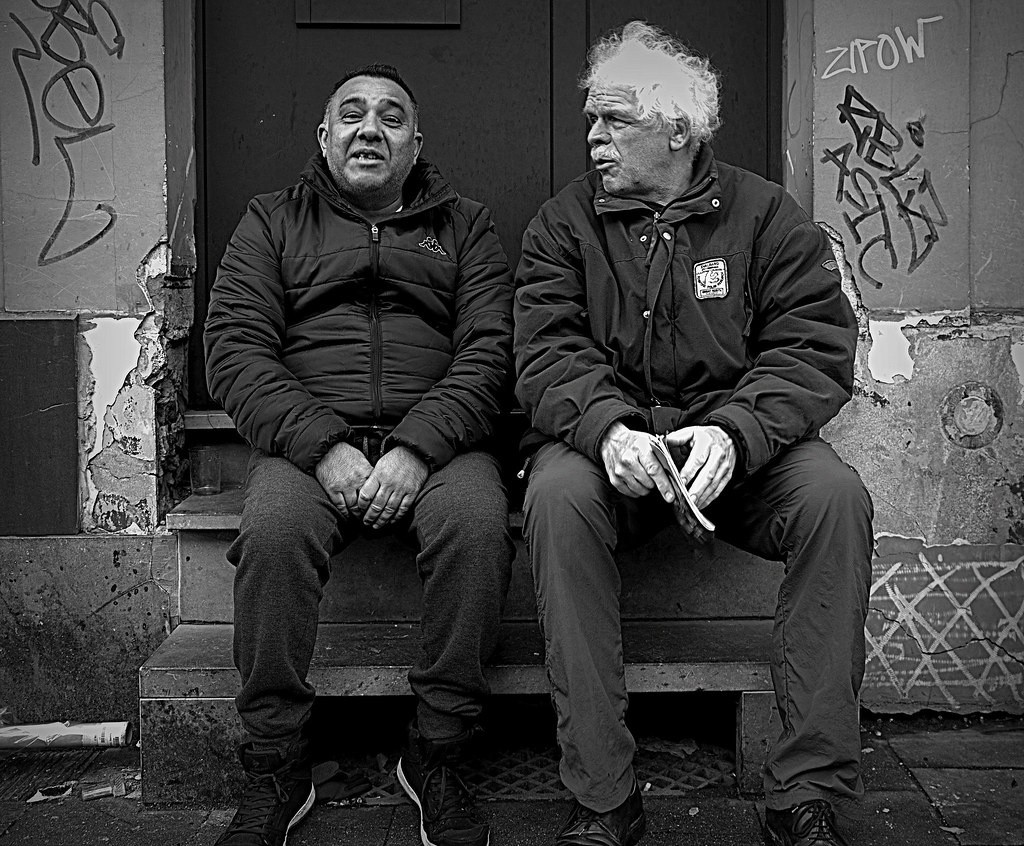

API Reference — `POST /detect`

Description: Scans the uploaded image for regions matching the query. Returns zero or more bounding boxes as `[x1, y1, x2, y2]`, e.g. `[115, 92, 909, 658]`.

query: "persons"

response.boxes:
[200, 60, 517, 846]
[511, 19, 875, 846]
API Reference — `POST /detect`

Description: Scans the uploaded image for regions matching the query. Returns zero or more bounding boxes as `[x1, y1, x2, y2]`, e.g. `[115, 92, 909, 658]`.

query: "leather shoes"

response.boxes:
[556, 778, 648, 846]
[764, 797, 844, 846]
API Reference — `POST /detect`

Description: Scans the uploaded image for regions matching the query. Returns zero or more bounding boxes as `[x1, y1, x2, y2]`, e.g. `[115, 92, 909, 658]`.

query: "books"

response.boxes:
[647, 429, 715, 551]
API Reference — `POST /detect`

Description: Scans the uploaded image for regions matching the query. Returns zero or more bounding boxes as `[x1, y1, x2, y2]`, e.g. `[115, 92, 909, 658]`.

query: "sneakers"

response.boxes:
[214, 738, 317, 846]
[398, 736, 495, 846]
